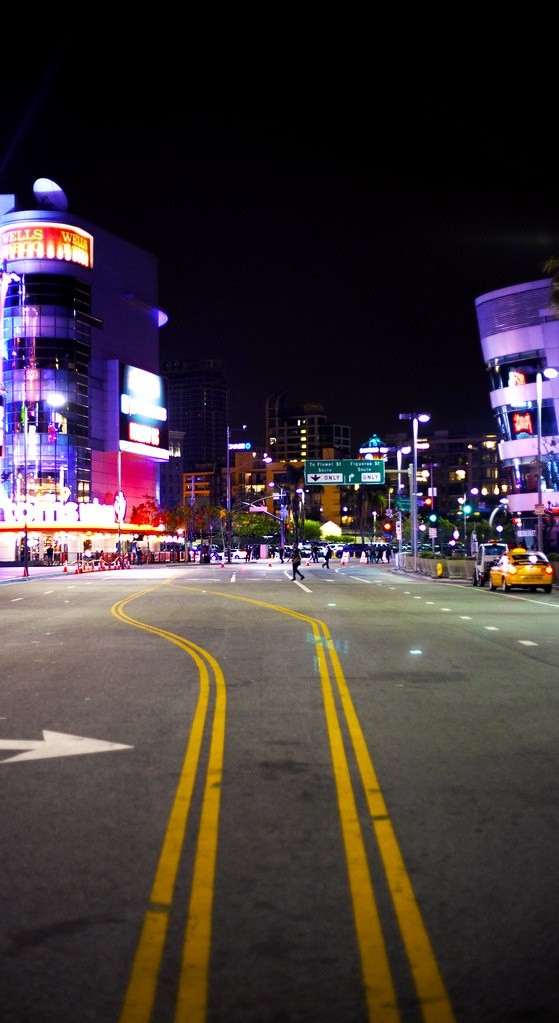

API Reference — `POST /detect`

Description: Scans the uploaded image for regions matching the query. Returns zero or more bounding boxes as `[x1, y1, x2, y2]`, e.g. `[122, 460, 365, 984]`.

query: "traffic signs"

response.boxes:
[304, 459, 386, 485]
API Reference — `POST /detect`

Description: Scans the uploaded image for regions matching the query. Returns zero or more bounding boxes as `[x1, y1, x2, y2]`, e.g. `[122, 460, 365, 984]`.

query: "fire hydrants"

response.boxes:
[436, 563, 443, 576]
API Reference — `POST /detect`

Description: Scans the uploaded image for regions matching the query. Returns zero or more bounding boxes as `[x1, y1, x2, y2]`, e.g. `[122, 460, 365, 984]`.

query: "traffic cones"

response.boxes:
[74, 563, 79, 574]
[23, 568, 29, 577]
[127, 561, 131, 569]
[220, 557, 369, 568]
[114, 561, 120, 570]
[63, 562, 68, 572]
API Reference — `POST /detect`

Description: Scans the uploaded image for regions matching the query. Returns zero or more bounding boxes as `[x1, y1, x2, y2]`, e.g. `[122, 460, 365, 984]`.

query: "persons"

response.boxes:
[47, 545, 53, 566]
[145, 547, 150, 564]
[309, 543, 322, 563]
[322, 544, 333, 569]
[136, 546, 143, 565]
[279, 545, 284, 564]
[95, 550, 104, 566]
[366, 543, 391, 563]
[287, 546, 305, 581]
[246, 544, 251, 562]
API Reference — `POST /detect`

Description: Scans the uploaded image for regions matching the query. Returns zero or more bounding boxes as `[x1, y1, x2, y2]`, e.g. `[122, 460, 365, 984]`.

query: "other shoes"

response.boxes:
[322, 564, 324, 568]
[300, 575, 304, 580]
[327, 567, 330, 569]
[280, 560, 284, 563]
[291, 577, 296, 581]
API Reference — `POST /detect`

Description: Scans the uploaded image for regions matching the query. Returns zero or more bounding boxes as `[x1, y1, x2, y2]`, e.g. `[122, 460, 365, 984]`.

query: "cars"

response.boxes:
[285, 542, 370, 559]
[489, 548, 553, 594]
[223, 549, 247, 559]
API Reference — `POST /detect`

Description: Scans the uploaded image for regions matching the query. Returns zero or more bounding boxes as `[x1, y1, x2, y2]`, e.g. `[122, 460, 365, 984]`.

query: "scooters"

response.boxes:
[472, 543, 509, 588]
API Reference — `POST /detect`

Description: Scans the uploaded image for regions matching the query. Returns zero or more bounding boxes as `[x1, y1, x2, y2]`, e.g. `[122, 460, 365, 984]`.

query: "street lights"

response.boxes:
[24, 395, 65, 577]
[400, 413, 431, 573]
[269, 482, 283, 499]
[296, 489, 305, 534]
[536, 367, 558, 552]
[389, 446, 411, 488]
[227, 458, 272, 530]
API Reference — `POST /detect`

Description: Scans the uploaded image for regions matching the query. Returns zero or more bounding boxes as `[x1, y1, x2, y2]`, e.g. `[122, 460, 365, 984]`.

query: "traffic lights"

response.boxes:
[464, 501, 472, 514]
[430, 510, 438, 528]
